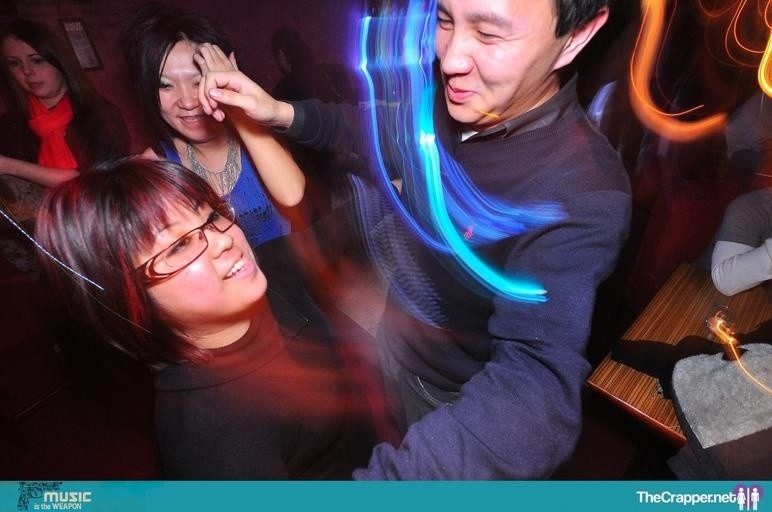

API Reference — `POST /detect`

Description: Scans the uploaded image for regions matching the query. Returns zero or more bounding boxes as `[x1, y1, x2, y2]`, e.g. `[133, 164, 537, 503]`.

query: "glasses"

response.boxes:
[132, 202, 236, 291]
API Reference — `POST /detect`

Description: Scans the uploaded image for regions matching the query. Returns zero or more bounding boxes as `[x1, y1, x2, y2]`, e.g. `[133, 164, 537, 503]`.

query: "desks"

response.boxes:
[588, 248, 771, 459]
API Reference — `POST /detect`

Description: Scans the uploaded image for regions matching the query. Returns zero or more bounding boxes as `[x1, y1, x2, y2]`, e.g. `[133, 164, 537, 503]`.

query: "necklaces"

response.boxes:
[186, 128, 239, 202]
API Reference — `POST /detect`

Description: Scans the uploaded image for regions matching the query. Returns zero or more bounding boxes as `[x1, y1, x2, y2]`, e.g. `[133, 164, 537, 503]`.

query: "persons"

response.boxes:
[195, 0, 635, 480]
[33, 157, 406, 483]
[127, 5, 310, 244]
[3, 12, 127, 192]
[260, 28, 379, 266]
[710, 183, 772, 299]
